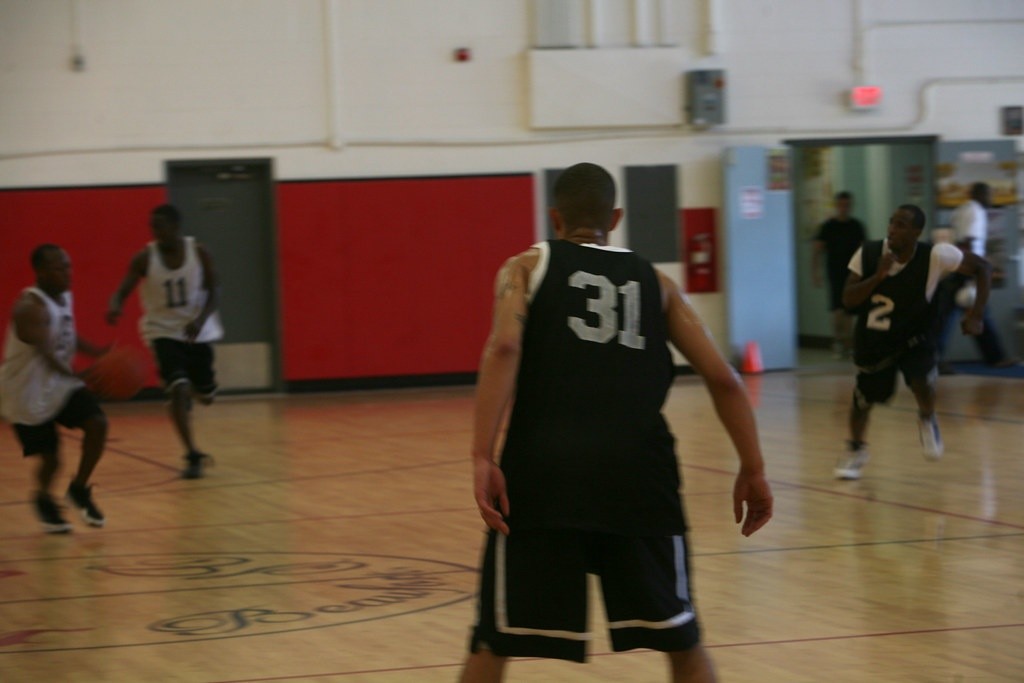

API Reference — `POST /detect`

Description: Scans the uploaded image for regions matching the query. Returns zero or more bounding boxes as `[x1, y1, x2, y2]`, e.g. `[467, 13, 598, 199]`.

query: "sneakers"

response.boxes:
[66, 483, 106, 524]
[31, 492, 69, 531]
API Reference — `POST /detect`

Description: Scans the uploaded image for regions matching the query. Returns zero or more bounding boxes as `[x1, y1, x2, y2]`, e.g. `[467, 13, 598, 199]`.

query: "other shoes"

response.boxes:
[919, 418, 942, 461]
[836, 447, 869, 481]
[182, 452, 214, 478]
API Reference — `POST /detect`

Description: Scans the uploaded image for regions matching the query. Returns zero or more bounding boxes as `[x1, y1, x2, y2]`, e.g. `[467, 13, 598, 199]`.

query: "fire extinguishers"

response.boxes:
[687, 232, 712, 292]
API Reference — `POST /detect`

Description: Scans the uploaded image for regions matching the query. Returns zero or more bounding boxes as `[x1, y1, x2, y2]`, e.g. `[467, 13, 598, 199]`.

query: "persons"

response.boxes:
[459, 162, 773, 683]
[932, 182, 1024, 371]
[833, 204, 994, 479]
[108, 205, 225, 479]
[811, 191, 867, 361]
[0, 244, 113, 533]
